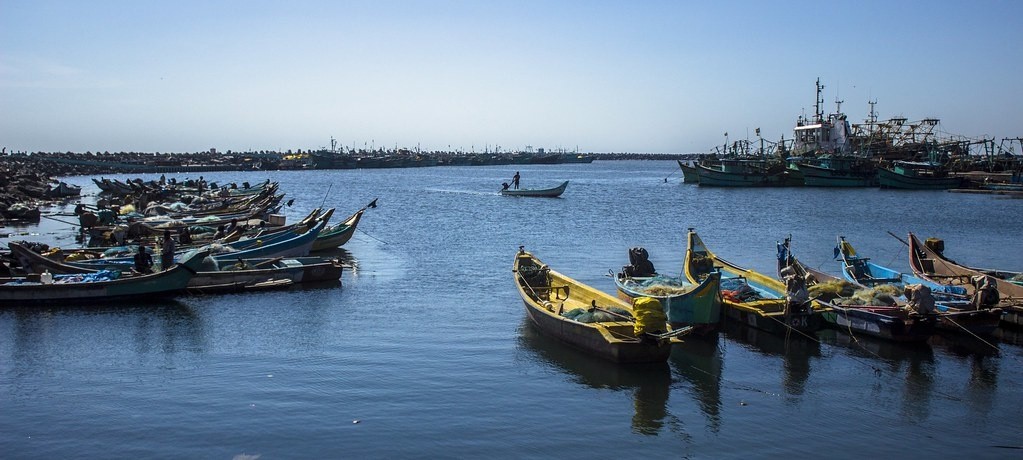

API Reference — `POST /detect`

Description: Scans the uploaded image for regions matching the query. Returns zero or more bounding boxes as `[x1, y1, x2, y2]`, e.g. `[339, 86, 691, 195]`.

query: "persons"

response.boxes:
[213, 226, 225, 240]
[513, 171, 520, 189]
[134, 246, 154, 274]
[258, 221, 267, 231]
[160, 231, 175, 270]
[227, 219, 237, 232]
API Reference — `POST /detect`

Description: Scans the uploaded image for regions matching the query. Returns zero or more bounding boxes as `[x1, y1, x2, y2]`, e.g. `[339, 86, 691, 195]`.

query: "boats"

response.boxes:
[236, 135, 597, 171]
[606, 246, 722, 339]
[0, 177, 378, 308]
[776, 234, 941, 344]
[501, 181, 570, 197]
[51, 182, 82, 197]
[676, 76, 1023, 199]
[512, 244, 695, 370]
[833, 230, 1023, 334]
[684, 228, 832, 359]
